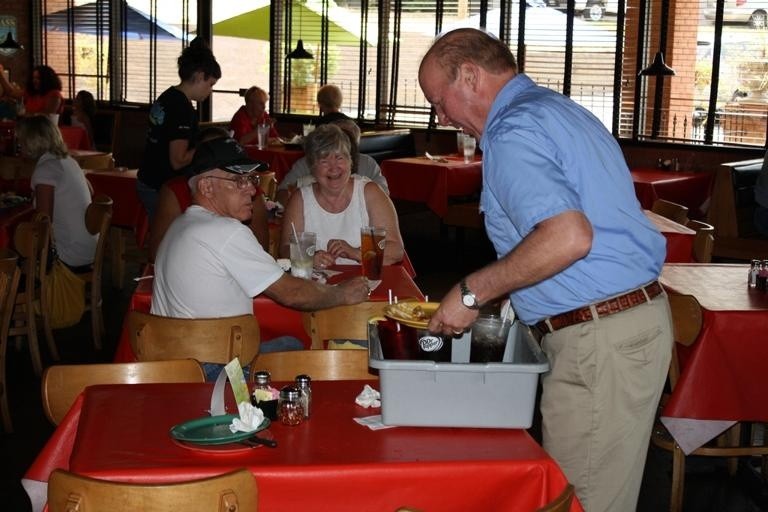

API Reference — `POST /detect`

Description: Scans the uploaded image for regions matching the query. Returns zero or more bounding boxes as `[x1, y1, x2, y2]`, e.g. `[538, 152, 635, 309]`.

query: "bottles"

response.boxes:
[293, 373, 314, 417]
[277, 387, 303, 427]
[657, 156, 681, 172]
[251, 372, 278, 421]
[747, 258, 768, 288]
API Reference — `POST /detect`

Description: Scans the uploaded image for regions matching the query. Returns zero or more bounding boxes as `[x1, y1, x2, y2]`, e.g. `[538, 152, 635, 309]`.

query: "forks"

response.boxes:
[424, 152, 448, 164]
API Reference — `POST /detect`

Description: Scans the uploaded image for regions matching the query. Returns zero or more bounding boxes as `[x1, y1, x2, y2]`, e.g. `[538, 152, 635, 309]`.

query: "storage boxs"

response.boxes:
[364, 310, 553, 433]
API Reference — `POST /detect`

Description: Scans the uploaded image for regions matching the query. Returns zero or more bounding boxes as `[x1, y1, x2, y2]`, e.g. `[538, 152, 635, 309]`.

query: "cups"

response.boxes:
[457, 132, 470, 159]
[257, 123, 271, 150]
[288, 231, 317, 279]
[377, 322, 421, 360]
[468, 317, 511, 363]
[462, 135, 476, 163]
[361, 227, 388, 282]
[302, 123, 315, 136]
[418, 329, 452, 362]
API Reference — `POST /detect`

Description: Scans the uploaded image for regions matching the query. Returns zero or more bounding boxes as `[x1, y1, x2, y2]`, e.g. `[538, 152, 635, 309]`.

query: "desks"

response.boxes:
[241, 134, 305, 172]
[378, 151, 483, 249]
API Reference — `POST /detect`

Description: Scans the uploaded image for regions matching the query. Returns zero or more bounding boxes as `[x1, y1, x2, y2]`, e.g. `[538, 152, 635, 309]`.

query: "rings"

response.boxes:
[320, 263, 324, 267]
[450, 328, 465, 337]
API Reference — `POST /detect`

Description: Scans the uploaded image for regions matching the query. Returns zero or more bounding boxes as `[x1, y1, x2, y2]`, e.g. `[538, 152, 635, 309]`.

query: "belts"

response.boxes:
[532, 281, 663, 336]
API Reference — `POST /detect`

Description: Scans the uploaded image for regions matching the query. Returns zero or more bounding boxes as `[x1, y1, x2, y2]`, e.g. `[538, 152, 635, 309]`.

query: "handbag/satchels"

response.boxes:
[40, 261, 86, 330]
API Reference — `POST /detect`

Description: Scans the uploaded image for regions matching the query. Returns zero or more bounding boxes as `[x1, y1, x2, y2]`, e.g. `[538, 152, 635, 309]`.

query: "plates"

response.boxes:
[174, 430, 279, 452]
[169, 411, 272, 445]
[281, 140, 303, 147]
[380, 301, 443, 329]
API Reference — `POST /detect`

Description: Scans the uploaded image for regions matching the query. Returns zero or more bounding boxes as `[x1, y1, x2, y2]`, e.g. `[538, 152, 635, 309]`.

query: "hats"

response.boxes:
[185, 135, 269, 180]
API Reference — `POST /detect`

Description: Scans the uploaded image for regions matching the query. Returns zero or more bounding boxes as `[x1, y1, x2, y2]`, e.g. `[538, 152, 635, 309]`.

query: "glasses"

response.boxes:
[201, 172, 261, 190]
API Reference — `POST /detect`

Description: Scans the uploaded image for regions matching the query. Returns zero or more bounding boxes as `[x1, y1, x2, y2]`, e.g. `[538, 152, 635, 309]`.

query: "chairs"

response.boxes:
[55, 191, 116, 350]
[126, 305, 263, 372]
[628, 158, 766, 264]
[0, 250, 23, 429]
[9, 106, 236, 250]
[359, 126, 417, 172]
[270, 111, 378, 140]
[39, 358, 206, 428]
[299, 300, 445, 351]
[392, 483, 578, 512]
[646, 290, 766, 512]
[11, 209, 66, 378]
[43, 460, 263, 512]
[247, 343, 376, 382]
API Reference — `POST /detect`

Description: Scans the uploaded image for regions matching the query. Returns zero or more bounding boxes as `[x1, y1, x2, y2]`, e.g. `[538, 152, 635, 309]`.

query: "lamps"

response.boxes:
[633, 49, 677, 79]
[0, 0, 31, 54]
[282, 0, 316, 63]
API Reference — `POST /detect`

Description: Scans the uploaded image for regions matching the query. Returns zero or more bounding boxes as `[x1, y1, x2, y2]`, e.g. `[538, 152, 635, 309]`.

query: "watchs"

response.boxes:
[459, 275, 480, 313]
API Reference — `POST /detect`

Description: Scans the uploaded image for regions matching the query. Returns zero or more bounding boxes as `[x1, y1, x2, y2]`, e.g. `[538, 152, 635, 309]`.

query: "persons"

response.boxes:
[61, 90, 110, 151]
[279, 124, 404, 270]
[136, 40, 221, 226]
[156, 125, 265, 244]
[277, 118, 390, 208]
[2, 65, 64, 116]
[57, 105, 96, 167]
[149, 133, 372, 386]
[16, 116, 100, 273]
[417, 24, 675, 510]
[59, 98, 73, 115]
[315, 85, 352, 128]
[230, 86, 284, 145]
